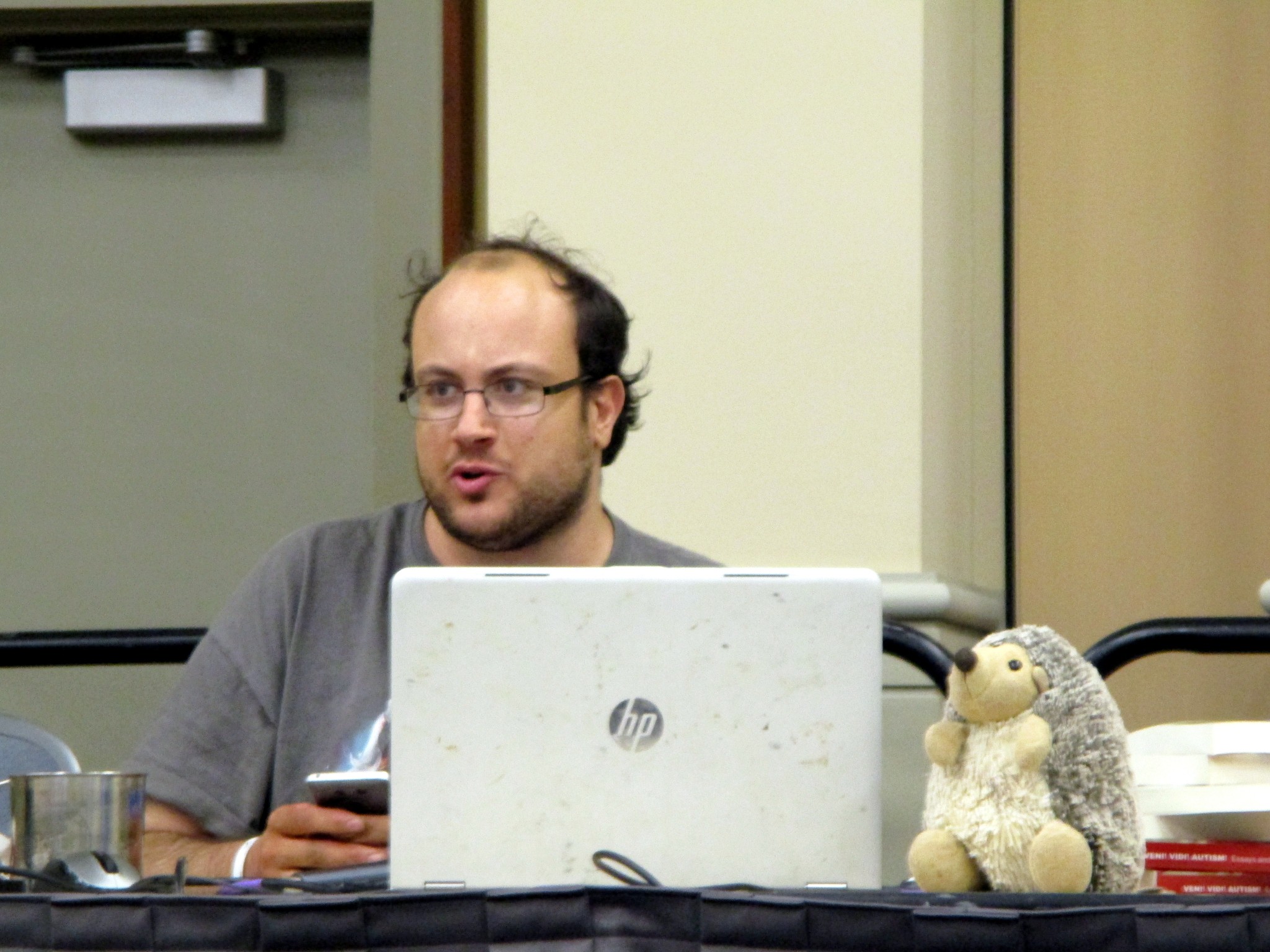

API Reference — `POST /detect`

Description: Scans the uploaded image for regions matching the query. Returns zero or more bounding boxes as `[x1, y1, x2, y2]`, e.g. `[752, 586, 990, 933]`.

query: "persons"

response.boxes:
[104, 238, 727, 893]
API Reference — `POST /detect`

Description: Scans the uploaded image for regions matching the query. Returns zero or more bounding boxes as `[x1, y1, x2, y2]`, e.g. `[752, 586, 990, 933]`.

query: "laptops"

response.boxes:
[389, 568, 885, 893]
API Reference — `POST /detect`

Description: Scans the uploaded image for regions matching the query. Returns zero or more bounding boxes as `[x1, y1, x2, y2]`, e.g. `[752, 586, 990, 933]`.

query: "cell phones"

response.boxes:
[306, 771, 391, 841]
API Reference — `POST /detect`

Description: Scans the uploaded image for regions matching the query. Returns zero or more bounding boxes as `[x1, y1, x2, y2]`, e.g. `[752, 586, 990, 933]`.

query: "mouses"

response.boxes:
[28, 850, 140, 890]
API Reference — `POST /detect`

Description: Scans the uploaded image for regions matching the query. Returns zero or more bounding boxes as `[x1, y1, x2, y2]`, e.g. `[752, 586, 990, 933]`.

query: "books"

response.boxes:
[1126, 720, 1270, 900]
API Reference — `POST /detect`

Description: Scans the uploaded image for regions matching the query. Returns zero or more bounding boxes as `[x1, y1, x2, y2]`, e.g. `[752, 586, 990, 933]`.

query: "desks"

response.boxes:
[0, 885, 1270, 952]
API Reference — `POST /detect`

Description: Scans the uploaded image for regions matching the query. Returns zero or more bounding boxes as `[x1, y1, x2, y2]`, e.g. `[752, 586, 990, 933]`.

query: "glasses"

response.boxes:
[399, 374, 594, 422]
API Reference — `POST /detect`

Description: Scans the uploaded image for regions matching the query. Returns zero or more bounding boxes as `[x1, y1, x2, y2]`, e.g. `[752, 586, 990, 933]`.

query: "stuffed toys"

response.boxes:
[907, 623, 1144, 899]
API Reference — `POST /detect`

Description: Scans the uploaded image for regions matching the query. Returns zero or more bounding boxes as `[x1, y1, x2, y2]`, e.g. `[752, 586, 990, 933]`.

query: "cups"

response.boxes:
[0, 771, 147, 893]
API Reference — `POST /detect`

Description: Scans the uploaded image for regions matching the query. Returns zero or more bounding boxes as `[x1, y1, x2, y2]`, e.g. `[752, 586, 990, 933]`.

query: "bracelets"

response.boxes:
[231, 836, 258, 879]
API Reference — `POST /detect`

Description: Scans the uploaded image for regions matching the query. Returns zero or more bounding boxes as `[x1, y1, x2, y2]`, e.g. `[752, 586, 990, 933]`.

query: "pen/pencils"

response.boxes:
[215, 878, 288, 893]
